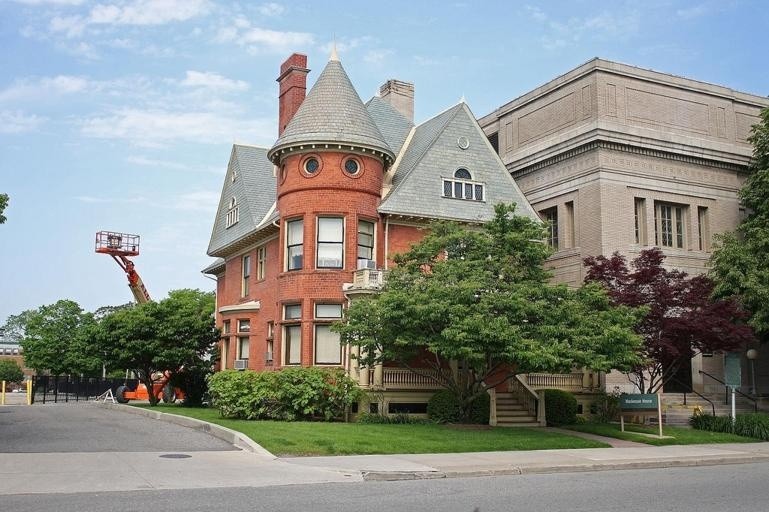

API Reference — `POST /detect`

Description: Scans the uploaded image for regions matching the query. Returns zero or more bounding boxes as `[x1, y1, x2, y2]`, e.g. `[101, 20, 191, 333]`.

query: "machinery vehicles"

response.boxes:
[94, 230, 206, 403]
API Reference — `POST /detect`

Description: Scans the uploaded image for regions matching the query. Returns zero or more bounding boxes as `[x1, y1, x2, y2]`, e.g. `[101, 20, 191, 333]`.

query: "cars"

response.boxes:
[18, 387, 27, 393]
